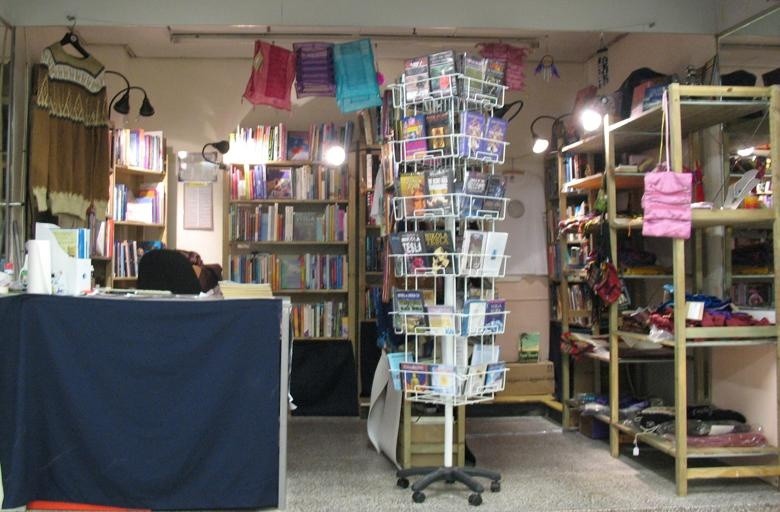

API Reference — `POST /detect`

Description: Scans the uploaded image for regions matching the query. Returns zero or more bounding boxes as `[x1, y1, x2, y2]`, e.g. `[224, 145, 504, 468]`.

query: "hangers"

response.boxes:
[42, 16, 105, 71]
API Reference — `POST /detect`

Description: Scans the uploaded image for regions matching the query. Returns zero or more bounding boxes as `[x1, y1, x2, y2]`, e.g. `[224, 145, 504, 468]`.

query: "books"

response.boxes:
[389, 230, 508, 277]
[387, 344, 506, 397]
[360, 73, 405, 145]
[404, 50, 504, 108]
[395, 287, 506, 336]
[217, 279, 274, 301]
[540, 149, 657, 329]
[231, 118, 353, 339]
[365, 140, 404, 226]
[400, 110, 507, 166]
[399, 166, 506, 226]
[49, 227, 93, 261]
[364, 228, 394, 320]
[106, 126, 170, 281]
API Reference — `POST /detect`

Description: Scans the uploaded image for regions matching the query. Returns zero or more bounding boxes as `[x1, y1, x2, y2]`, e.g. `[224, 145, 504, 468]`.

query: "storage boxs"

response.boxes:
[495, 359, 555, 396]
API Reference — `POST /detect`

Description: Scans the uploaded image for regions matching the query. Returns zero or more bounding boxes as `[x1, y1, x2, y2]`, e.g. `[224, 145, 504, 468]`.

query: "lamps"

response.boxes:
[530, 105, 601, 154]
[105, 71, 155, 121]
[202, 141, 229, 171]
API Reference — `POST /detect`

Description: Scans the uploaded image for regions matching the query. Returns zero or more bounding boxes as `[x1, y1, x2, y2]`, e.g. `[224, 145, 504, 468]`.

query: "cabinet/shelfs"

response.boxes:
[92, 121, 168, 291]
[222, 139, 385, 420]
[557, 82, 780, 497]
